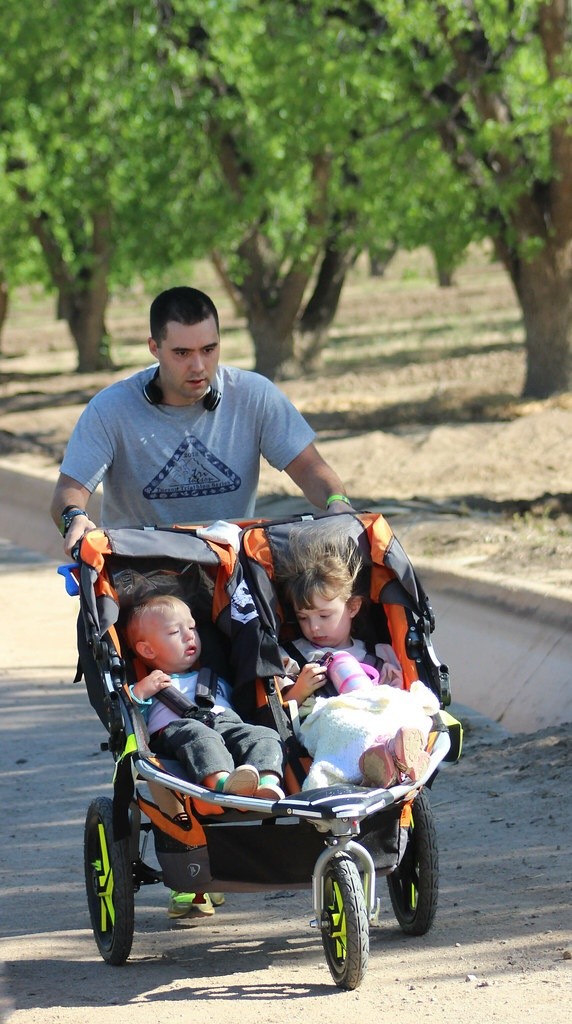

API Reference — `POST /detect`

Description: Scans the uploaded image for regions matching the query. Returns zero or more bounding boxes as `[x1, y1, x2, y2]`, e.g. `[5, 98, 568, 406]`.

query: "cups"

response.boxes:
[322, 651, 377, 694]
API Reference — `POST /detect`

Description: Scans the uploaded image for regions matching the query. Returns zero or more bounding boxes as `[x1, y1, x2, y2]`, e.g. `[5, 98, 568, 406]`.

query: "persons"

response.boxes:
[52, 286, 358, 925]
[275, 542, 431, 787]
[127, 595, 287, 803]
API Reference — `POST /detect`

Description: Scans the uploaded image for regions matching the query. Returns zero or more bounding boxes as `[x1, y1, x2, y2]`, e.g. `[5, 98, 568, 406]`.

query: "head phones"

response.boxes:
[142, 366, 222, 411]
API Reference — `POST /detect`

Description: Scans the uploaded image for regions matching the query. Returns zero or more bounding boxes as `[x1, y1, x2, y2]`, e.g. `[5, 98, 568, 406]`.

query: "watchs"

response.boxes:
[59, 510, 88, 537]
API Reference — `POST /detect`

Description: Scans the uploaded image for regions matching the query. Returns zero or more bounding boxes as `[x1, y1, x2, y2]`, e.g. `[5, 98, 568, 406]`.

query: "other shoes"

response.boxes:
[222, 764, 259, 797]
[257, 784, 285, 800]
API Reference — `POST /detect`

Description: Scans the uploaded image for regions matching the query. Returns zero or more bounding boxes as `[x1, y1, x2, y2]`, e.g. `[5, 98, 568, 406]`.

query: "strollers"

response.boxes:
[70, 508, 466, 989]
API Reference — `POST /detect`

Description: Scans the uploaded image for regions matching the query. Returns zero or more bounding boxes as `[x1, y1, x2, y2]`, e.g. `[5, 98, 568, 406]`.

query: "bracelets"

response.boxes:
[325, 495, 352, 511]
[61, 505, 81, 516]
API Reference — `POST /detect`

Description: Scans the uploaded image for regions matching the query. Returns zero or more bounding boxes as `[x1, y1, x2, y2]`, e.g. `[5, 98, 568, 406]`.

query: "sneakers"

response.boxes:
[375, 727, 430, 782]
[167, 888, 215, 919]
[359, 743, 402, 788]
[209, 892, 225, 904]
